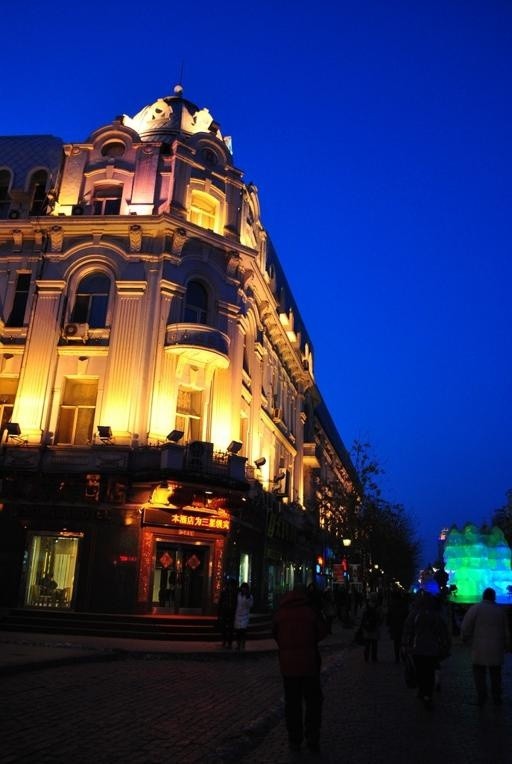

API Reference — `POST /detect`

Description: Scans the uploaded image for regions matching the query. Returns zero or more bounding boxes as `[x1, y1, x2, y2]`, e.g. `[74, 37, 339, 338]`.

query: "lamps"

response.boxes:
[254, 457, 265, 468]
[273, 472, 285, 485]
[4, 423, 28, 444]
[165, 430, 183, 445]
[97, 426, 114, 447]
[225, 441, 243, 456]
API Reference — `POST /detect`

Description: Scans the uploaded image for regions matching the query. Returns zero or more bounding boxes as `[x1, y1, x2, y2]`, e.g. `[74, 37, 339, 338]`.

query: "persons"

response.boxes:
[38, 573, 66, 608]
[388, 592, 448, 696]
[305, 583, 385, 663]
[216, 577, 254, 648]
[461, 588, 511, 707]
[272, 582, 330, 755]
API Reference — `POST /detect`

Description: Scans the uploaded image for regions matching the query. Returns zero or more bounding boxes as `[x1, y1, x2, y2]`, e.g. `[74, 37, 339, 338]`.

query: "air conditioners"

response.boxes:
[63, 323, 89, 340]
[186, 441, 213, 474]
[72, 204, 95, 215]
[7, 209, 30, 219]
[274, 408, 281, 420]
[278, 468, 290, 494]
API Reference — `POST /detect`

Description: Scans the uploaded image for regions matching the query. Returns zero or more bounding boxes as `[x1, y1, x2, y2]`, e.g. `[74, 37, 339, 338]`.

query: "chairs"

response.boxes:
[32, 585, 71, 607]
